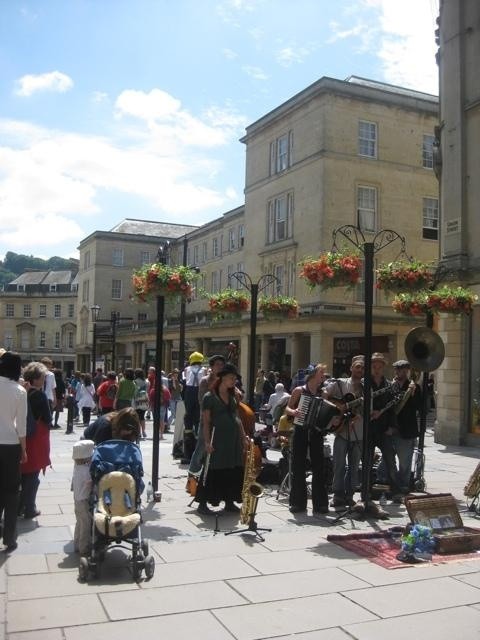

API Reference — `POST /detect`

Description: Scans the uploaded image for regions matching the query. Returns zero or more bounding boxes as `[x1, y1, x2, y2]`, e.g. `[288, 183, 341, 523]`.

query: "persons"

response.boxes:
[182, 351, 207, 431]
[273, 396, 295, 464]
[71, 439, 95, 556]
[263, 383, 289, 431]
[0, 351, 27, 549]
[166, 368, 183, 430]
[147, 367, 171, 440]
[113, 368, 137, 410]
[79, 373, 95, 427]
[80, 406, 140, 444]
[326, 355, 372, 507]
[41, 357, 57, 407]
[361, 353, 401, 505]
[196, 363, 249, 517]
[96, 371, 118, 414]
[376, 360, 420, 497]
[16, 362, 52, 519]
[185, 354, 225, 494]
[131, 368, 148, 438]
[284, 361, 341, 513]
[50, 369, 65, 428]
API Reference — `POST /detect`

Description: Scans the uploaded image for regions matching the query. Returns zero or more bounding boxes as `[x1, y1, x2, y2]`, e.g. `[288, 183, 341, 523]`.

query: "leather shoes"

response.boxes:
[197, 506, 213, 515]
[289, 505, 303, 513]
[313, 507, 328, 514]
[24, 509, 41, 519]
[224, 504, 241, 512]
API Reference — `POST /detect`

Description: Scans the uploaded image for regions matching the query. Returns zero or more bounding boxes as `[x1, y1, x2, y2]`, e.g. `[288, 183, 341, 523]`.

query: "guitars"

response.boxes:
[325, 385, 392, 435]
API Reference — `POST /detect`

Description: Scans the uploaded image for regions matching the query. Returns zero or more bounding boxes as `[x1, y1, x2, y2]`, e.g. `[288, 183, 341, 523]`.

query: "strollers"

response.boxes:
[77, 438, 155, 585]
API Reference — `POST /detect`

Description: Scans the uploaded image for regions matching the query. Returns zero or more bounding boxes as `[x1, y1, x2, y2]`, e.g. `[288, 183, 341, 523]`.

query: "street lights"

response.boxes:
[88, 304, 101, 375]
[90, 304, 101, 375]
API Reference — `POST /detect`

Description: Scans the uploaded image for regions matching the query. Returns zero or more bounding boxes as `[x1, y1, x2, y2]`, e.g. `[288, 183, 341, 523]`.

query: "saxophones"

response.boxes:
[240, 437, 265, 526]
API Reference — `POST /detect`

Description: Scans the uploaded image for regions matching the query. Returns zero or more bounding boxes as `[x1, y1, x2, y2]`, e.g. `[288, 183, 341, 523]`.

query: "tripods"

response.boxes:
[222, 512, 272, 543]
[332, 381, 368, 529]
[276, 427, 314, 501]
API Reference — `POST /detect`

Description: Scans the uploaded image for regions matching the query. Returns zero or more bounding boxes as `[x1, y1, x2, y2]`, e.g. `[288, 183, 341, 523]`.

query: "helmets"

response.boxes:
[189, 351, 205, 365]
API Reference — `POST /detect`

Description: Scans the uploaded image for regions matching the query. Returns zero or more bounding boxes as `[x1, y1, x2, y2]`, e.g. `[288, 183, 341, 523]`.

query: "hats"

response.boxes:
[392, 360, 410, 369]
[371, 352, 387, 365]
[216, 363, 241, 381]
[72, 439, 95, 459]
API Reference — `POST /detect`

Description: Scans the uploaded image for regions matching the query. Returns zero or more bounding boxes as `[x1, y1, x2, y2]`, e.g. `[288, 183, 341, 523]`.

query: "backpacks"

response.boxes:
[133, 379, 148, 405]
[104, 384, 118, 401]
[26, 390, 39, 437]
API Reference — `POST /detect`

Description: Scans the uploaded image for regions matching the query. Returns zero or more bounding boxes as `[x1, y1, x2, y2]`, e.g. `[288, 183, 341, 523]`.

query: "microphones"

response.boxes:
[328, 378, 340, 384]
